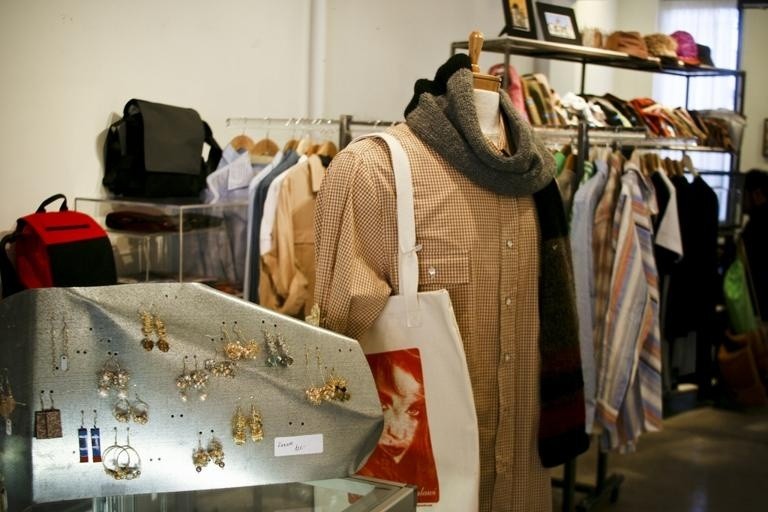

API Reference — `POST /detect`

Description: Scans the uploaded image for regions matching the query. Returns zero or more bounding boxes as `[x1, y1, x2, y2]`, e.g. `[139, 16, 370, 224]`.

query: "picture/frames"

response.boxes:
[535, 1, 583, 46]
[502, 0, 538, 39]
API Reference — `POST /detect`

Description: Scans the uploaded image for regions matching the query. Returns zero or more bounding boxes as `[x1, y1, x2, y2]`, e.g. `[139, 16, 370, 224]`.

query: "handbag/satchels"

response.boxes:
[313, 290, 482, 511]
[12, 194, 117, 291]
[716, 324, 767, 407]
[103, 98, 220, 203]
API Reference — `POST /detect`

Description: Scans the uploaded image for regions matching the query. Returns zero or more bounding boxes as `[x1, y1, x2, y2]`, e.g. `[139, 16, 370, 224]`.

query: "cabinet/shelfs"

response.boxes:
[450, 36, 747, 418]
[73, 196, 250, 300]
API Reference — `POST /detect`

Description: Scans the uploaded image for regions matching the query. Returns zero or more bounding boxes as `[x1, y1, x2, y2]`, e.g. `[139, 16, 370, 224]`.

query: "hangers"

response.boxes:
[219, 115, 405, 175]
[532, 124, 699, 184]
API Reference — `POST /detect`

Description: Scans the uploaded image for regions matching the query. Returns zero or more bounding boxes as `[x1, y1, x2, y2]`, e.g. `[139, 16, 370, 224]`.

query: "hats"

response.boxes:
[608, 30, 714, 68]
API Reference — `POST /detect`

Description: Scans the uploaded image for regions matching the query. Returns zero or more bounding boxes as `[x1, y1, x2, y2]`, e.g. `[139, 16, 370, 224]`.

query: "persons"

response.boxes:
[310, 30, 591, 512]
[357, 347, 440, 506]
